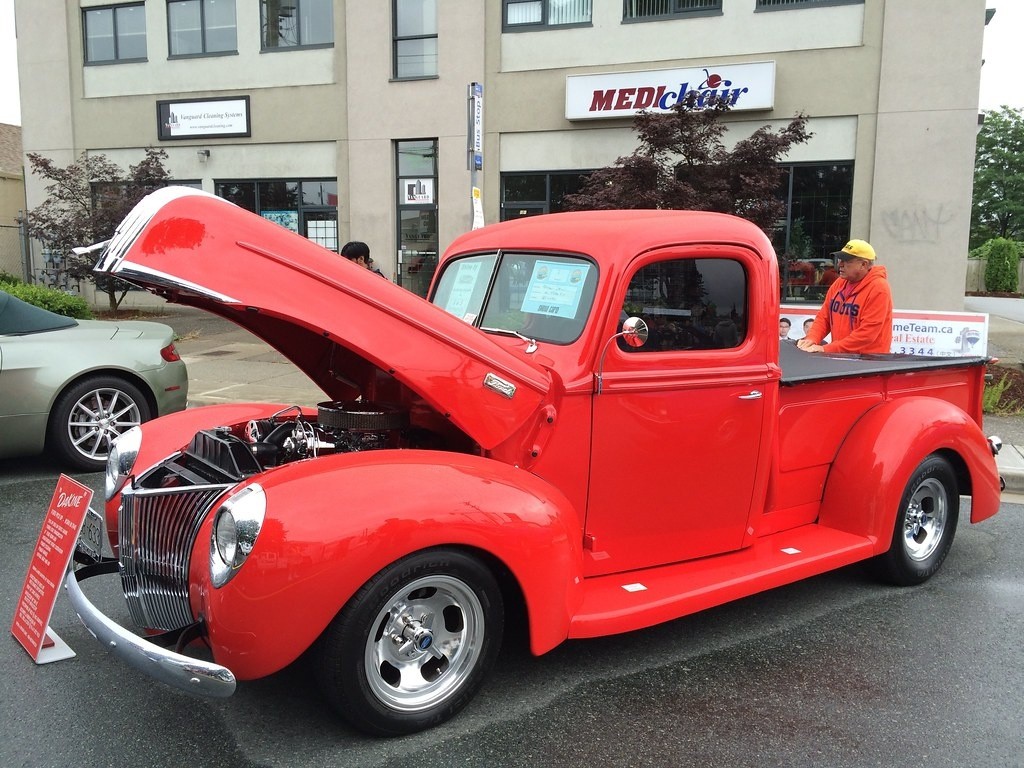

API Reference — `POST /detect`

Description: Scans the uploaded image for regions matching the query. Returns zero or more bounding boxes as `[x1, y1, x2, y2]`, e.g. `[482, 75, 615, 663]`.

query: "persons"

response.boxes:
[797, 239, 892, 353]
[779, 318, 828, 347]
[712, 306, 738, 349]
[342, 241, 384, 278]
[815, 261, 842, 292]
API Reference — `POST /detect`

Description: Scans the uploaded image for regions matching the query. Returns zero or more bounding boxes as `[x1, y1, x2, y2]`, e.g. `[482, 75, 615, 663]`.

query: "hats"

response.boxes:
[830, 239, 875, 261]
[341, 241, 373, 264]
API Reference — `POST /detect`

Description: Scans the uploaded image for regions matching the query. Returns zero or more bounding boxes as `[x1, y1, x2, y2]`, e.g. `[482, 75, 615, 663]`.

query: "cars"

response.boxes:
[57, 184, 1007, 737]
[777, 257, 834, 286]
[0, 289, 189, 474]
